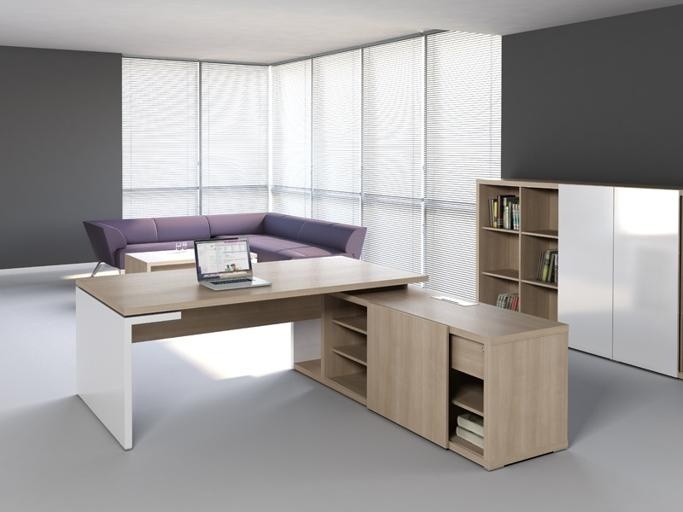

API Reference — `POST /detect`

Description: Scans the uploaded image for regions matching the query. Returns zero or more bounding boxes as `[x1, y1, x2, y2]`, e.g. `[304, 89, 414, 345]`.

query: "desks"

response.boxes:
[74, 254, 570, 471]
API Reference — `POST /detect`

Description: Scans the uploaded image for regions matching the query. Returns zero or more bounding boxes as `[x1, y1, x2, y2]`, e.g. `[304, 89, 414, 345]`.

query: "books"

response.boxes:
[455, 425, 483, 449]
[488, 192, 522, 231]
[457, 413, 484, 439]
[495, 293, 519, 311]
[534, 248, 559, 285]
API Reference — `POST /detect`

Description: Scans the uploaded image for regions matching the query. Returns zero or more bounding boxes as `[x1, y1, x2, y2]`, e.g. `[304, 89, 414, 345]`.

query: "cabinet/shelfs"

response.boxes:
[475, 177, 683, 380]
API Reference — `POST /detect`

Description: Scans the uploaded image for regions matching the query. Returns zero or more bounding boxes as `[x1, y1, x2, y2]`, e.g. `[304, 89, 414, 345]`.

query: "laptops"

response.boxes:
[194, 238, 272, 291]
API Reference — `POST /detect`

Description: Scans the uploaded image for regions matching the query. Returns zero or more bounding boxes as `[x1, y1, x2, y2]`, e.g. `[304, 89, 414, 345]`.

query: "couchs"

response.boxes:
[82, 211, 367, 277]
